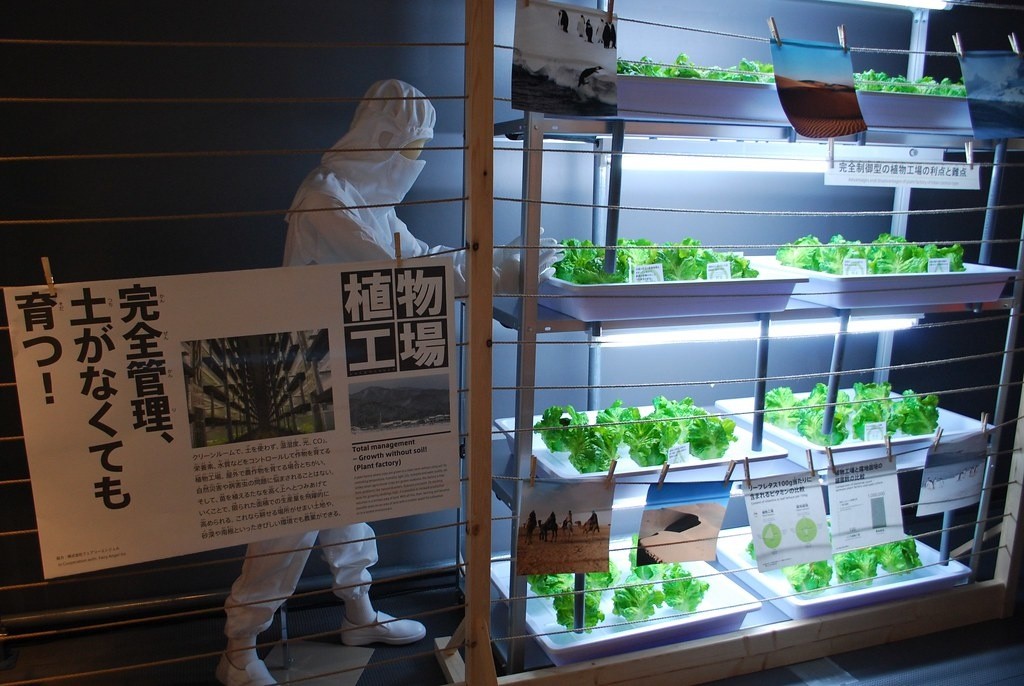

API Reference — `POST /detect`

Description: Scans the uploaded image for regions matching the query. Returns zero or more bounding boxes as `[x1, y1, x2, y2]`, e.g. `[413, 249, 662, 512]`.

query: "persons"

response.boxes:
[216, 76, 569, 686]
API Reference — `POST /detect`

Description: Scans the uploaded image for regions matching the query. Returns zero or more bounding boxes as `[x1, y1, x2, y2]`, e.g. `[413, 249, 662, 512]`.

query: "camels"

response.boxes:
[562, 512, 573, 541]
[524, 511, 558, 545]
[574, 513, 600, 540]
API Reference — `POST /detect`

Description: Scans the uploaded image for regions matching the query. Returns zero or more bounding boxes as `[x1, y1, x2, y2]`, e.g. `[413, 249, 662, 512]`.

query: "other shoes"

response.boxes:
[341, 611, 426, 645]
[216, 652, 278, 686]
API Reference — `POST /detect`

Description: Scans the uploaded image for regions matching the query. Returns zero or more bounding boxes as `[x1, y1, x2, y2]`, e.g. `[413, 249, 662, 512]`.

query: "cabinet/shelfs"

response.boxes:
[460, 58, 1024, 686]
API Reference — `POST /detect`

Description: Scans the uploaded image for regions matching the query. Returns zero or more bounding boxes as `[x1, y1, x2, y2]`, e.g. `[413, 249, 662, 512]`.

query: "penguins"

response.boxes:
[558, 9, 570, 33]
[595, 18, 616, 49]
[577, 15, 593, 44]
[577, 66, 604, 88]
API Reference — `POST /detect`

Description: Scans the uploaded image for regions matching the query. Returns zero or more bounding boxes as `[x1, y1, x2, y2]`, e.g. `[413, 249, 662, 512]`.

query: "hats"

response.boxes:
[321, 79, 436, 208]
